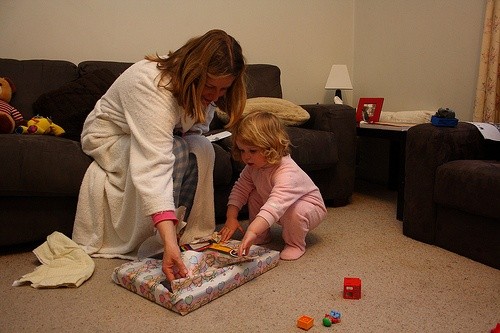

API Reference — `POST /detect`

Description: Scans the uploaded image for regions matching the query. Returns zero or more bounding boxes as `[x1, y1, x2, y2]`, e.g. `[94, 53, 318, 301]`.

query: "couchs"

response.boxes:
[0, 58, 355, 249]
[402, 121, 500, 270]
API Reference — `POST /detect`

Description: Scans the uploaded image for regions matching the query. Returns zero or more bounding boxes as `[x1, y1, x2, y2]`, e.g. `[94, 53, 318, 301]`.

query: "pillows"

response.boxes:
[32, 67, 116, 139]
[241, 96, 310, 126]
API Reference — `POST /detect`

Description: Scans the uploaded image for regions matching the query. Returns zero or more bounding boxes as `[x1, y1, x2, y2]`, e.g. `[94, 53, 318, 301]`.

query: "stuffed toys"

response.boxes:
[0, 78, 23, 134]
[15, 115, 65, 134]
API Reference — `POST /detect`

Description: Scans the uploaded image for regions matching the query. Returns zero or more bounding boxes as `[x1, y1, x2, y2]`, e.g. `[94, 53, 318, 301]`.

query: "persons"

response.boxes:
[217, 110, 329, 261]
[72, 29, 247, 283]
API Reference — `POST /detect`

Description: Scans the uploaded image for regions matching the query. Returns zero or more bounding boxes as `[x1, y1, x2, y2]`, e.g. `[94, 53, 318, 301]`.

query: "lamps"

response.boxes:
[324, 64, 353, 104]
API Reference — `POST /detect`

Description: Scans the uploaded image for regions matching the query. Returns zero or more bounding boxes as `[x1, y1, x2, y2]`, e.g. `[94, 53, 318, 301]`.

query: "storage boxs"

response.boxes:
[111, 232, 281, 316]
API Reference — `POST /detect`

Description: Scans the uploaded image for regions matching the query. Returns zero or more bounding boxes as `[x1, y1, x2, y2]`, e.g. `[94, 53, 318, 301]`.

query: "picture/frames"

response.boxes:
[356, 97, 384, 124]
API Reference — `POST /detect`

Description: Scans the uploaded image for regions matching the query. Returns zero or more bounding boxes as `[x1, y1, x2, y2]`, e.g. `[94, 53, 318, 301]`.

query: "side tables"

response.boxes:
[356, 123, 410, 221]
